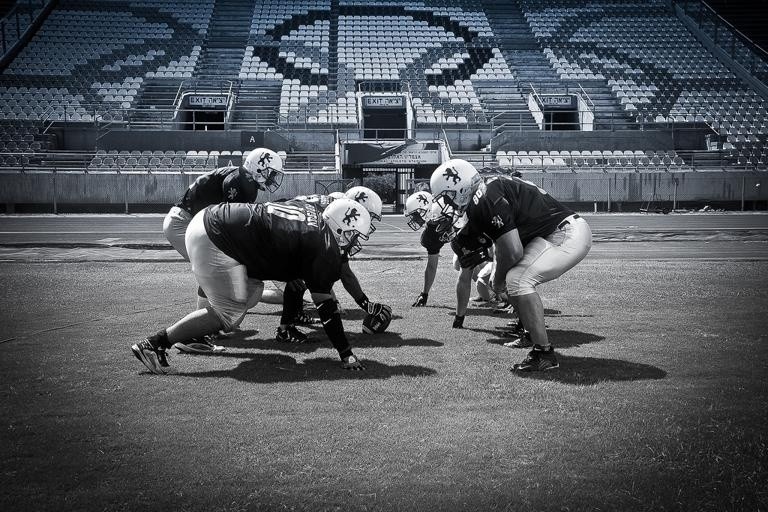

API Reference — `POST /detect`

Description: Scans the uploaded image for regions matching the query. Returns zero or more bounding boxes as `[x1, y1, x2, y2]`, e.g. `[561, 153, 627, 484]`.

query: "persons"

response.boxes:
[430, 159, 593, 372]
[130, 197, 371, 375]
[429, 196, 515, 328]
[269, 192, 346, 308]
[163, 148, 284, 355]
[272, 186, 391, 353]
[403, 190, 488, 308]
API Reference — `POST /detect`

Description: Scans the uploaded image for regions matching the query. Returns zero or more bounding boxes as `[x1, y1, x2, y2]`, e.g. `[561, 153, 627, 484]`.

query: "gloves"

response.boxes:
[333, 347, 367, 371]
[452, 314, 463, 326]
[356, 292, 389, 323]
[411, 290, 429, 307]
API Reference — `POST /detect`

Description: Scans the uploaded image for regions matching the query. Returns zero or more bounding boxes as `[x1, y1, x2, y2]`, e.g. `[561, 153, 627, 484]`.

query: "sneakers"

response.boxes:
[276, 311, 319, 347]
[511, 347, 560, 375]
[129, 336, 177, 377]
[468, 290, 515, 314]
[506, 316, 531, 347]
[180, 335, 228, 356]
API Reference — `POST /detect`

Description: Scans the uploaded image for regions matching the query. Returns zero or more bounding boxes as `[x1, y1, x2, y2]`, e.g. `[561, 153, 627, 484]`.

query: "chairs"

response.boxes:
[0, 1, 767, 173]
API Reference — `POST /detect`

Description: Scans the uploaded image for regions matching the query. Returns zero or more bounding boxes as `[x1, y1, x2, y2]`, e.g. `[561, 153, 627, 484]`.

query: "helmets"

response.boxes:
[428, 159, 484, 221]
[240, 145, 285, 194]
[404, 190, 468, 243]
[323, 183, 383, 256]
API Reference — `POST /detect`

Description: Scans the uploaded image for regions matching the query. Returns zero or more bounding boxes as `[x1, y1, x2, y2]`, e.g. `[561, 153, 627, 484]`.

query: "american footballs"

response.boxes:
[362, 305, 391, 335]
